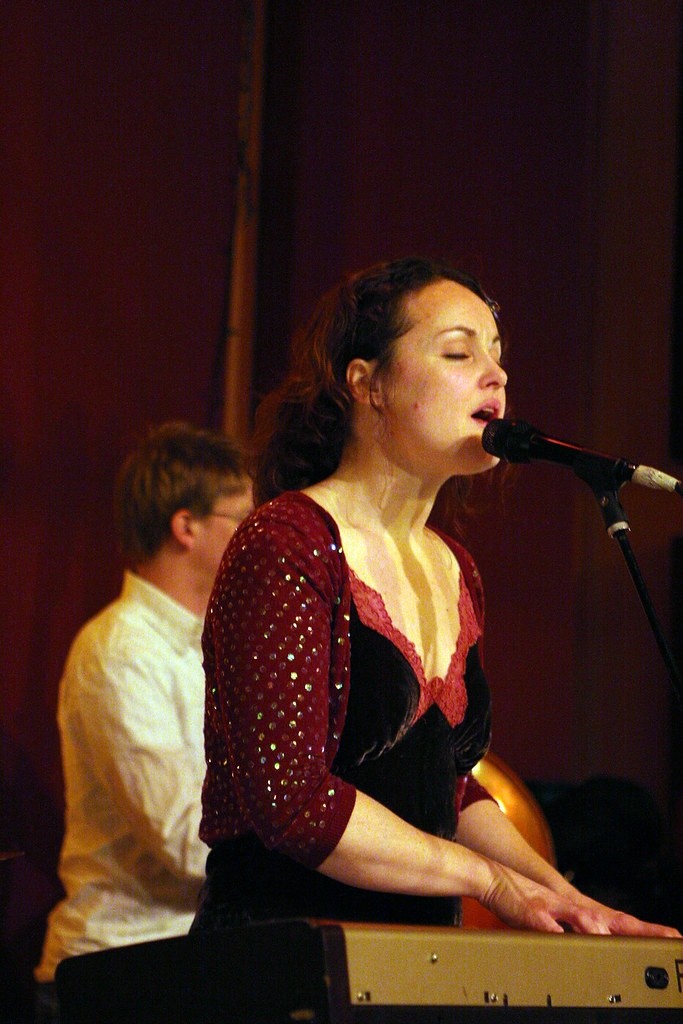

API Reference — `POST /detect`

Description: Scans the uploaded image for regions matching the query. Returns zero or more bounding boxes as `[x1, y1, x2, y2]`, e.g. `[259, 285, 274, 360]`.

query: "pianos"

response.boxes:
[50, 915, 683, 1024]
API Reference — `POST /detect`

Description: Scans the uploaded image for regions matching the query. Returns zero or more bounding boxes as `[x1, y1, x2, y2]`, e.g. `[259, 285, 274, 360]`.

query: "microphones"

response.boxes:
[482, 418, 683, 493]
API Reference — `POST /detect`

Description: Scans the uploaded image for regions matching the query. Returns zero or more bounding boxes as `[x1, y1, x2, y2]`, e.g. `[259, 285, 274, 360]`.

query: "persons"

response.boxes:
[34, 422, 254, 1023]
[176, 256, 682, 1024]
